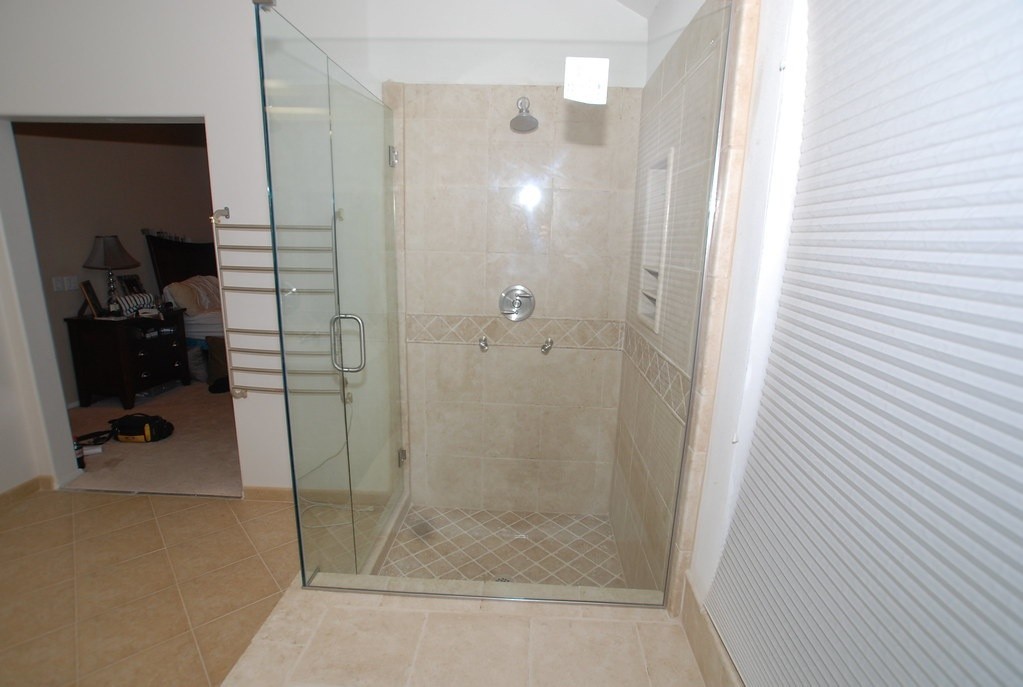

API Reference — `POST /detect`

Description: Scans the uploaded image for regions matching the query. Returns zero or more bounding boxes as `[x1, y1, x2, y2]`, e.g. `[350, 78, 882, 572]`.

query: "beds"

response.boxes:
[146, 235, 226, 348]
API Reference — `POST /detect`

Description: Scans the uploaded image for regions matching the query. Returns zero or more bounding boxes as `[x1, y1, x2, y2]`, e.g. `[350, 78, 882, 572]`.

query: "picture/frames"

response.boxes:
[77, 280, 104, 317]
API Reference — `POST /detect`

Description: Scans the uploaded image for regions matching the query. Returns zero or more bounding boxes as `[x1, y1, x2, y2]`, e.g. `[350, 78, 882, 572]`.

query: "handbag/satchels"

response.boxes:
[77, 413, 174, 446]
[117, 293, 165, 320]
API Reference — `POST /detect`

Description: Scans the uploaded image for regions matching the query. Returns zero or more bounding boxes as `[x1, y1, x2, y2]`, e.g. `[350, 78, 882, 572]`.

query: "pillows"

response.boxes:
[164, 276, 220, 317]
[186, 346, 208, 382]
[204, 336, 229, 383]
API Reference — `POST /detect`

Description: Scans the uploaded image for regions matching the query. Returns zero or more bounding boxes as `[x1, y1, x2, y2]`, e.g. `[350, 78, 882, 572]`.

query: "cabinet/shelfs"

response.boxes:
[63, 308, 192, 409]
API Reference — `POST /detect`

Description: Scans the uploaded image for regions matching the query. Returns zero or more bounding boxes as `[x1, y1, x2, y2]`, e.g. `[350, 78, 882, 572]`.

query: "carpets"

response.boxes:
[219, 570, 710, 687]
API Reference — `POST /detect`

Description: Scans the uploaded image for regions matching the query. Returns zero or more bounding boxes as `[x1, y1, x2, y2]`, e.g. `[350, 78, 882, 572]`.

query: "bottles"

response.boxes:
[73, 435, 86, 469]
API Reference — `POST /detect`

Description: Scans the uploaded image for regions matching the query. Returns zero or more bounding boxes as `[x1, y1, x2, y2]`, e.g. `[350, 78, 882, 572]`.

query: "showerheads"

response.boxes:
[509, 97, 539, 133]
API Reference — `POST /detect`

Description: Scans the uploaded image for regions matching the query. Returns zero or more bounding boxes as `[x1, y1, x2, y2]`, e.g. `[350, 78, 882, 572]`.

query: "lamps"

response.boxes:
[83, 235, 140, 315]
